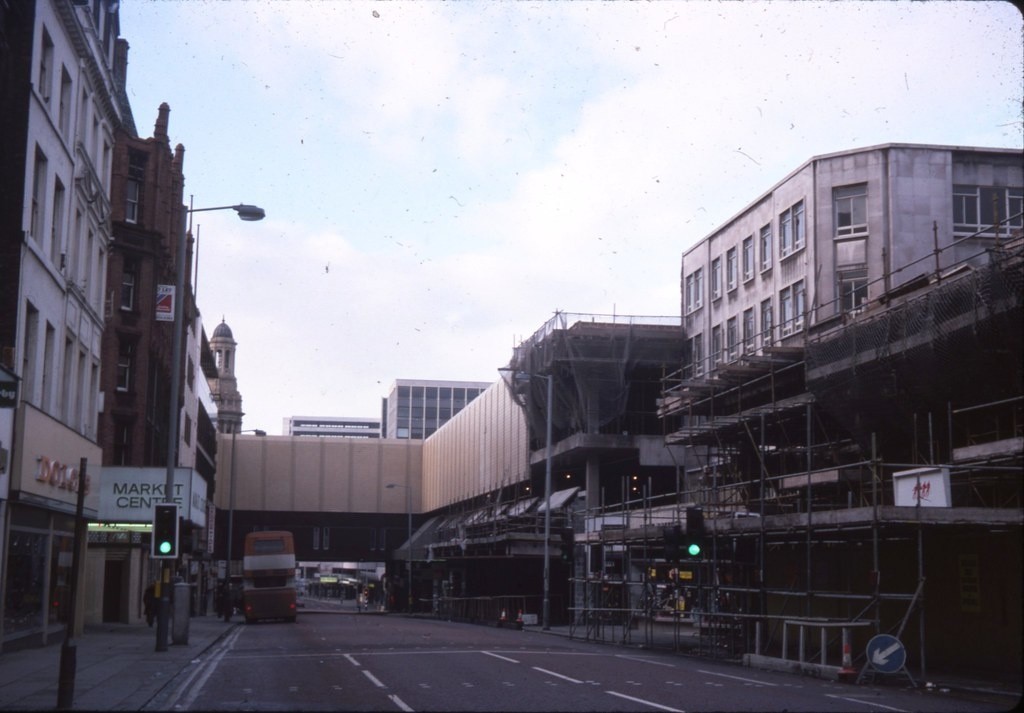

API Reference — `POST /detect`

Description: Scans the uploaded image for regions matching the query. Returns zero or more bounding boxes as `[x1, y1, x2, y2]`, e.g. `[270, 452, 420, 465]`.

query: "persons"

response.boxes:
[143, 575, 244, 628]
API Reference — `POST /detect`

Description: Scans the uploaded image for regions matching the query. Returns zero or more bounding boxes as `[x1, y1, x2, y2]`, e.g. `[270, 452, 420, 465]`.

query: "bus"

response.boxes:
[243, 530, 299, 623]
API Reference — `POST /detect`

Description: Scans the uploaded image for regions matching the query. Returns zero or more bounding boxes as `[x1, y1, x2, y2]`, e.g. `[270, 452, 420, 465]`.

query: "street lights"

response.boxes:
[221, 427, 266, 617]
[154, 200, 267, 650]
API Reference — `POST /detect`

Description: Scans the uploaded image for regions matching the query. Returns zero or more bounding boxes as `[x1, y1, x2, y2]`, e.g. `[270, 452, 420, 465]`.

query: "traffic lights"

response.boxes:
[686, 506, 704, 556]
[155, 506, 176, 555]
